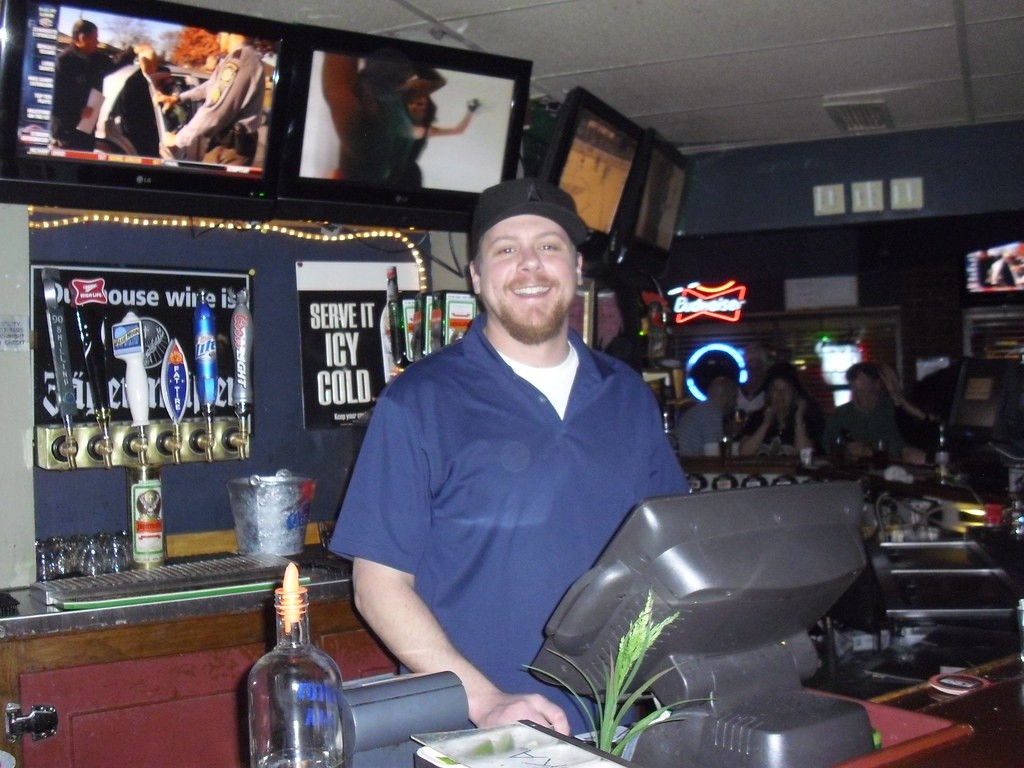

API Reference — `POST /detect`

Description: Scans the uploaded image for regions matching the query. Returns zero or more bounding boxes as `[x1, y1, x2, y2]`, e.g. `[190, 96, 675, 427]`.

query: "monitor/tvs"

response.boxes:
[0, 0, 692, 256]
[528, 480, 867, 694]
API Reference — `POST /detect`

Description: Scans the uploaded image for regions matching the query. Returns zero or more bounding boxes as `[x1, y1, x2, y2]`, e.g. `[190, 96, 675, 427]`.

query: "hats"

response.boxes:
[468, 177, 587, 261]
[846, 362, 877, 382]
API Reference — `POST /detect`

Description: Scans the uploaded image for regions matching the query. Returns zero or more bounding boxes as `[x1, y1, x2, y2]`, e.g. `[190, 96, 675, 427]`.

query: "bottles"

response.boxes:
[934, 423, 950, 479]
[389, 291, 478, 369]
[380, 265, 404, 384]
[885, 523, 941, 543]
[247, 563, 345, 768]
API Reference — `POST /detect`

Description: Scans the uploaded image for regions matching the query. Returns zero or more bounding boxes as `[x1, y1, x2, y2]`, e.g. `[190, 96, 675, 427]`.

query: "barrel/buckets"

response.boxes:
[225, 468, 311, 556]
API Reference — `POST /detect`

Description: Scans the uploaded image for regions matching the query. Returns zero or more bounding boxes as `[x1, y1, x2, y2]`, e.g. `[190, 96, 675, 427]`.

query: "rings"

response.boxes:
[767, 409, 769, 411]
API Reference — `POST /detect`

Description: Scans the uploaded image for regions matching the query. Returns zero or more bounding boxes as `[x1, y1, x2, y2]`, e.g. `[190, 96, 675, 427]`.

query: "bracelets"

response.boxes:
[794, 424, 805, 427]
[925, 455, 937, 467]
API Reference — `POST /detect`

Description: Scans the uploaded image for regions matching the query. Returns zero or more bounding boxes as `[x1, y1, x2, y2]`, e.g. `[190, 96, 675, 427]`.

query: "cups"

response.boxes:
[33, 529, 130, 583]
[703, 442, 720, 457]
[800, 447, 815, 467]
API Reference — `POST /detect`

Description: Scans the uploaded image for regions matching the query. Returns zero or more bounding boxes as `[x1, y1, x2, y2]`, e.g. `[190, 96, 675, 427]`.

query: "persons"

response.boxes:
[822, 362, 982, 467]
[50, 20, 263, 166]
[977, 250, 1024, 287]
[328, 179, 687, 738]
[739, 365, 826, 458]
[323, 54, 479, 186]
[677, 377, 738, 458]
